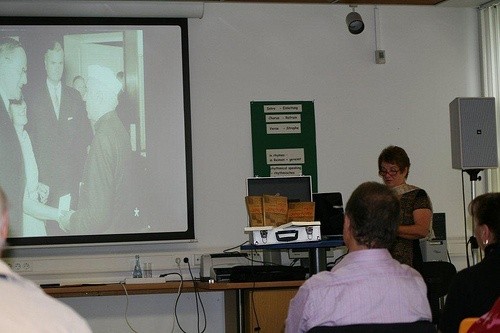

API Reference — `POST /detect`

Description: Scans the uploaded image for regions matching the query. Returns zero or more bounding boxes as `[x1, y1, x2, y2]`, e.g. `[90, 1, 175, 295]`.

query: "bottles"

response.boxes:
[133, 255, 142, 278]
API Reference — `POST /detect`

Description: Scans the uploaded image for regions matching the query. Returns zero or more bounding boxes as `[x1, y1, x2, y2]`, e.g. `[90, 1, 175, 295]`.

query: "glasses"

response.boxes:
[380, 166, 403, 176]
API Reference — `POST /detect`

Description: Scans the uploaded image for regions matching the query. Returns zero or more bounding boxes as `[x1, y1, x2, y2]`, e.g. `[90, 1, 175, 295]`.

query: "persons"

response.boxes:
[285, 180, 432, 333]
[0, 188, 93, 333]
[377, 147, 434, 267]
[0, 36, 132, 237]
[435, 192, 500, 333]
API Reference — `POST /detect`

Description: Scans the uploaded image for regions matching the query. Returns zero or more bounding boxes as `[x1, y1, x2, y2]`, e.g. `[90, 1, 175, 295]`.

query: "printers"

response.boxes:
[200, 253, 262, 282]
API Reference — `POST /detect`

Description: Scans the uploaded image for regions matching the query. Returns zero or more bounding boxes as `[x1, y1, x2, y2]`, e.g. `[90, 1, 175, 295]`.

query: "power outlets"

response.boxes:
[5, 260, 35, 272]
[172, 255, 192, 266]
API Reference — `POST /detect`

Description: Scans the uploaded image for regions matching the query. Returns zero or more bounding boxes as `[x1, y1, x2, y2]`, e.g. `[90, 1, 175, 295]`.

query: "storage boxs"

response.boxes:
[245, 174, 322, 246]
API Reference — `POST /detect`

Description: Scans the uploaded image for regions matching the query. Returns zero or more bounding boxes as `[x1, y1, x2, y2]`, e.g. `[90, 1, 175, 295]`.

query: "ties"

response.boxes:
[52, 86, 61, 120]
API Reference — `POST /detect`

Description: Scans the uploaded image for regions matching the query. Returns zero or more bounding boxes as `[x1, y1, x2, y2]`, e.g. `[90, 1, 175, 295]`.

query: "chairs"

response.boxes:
[308, 261, 456, 333]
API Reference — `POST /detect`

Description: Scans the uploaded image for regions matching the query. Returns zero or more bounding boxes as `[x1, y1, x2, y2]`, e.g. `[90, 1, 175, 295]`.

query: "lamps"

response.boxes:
[345, 4, 365, 35]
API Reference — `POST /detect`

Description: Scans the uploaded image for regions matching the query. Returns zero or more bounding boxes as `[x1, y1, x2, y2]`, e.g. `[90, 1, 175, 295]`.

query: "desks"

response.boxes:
[40, 240, 347, 333]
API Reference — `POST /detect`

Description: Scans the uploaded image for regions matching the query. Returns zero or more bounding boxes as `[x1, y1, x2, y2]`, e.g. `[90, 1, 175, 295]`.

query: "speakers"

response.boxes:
[448, 97, 498, 170]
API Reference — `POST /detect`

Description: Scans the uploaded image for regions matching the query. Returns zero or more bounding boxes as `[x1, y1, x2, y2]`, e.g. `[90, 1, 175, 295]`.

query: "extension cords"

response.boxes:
[125, 277, 166, 285]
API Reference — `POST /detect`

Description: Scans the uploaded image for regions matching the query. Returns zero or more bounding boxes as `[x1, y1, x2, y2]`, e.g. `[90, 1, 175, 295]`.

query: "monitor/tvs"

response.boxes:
[288, 247, 335, 268]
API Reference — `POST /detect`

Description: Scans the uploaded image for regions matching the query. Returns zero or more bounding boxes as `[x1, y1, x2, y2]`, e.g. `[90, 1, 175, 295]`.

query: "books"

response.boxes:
[245, 194, 316, 225]
